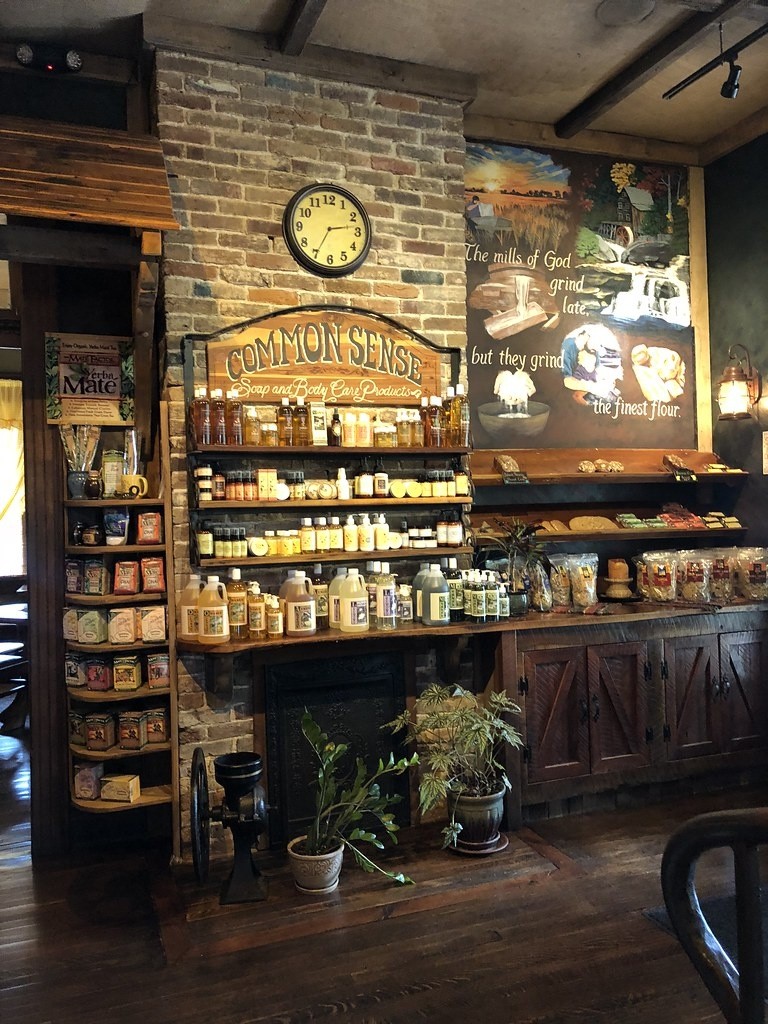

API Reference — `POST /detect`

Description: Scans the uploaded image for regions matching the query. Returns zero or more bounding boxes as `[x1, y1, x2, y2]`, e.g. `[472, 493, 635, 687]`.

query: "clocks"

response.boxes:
[282, 184, 372, 278]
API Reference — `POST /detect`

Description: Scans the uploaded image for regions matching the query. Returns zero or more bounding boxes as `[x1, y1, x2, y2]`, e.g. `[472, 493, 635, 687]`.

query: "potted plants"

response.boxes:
[377, 681, 527, 856]
[476, 516, 560, 618]
[286, 705, 421, 897]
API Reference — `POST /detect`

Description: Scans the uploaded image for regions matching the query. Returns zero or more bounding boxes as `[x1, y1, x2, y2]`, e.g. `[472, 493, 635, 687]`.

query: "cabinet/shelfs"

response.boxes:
[182, 304, 474, 566]
[62, 401, 180, 862]
[467, 448, 768, 827]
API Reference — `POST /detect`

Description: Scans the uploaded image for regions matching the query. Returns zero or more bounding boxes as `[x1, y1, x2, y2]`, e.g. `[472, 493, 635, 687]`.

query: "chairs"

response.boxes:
[660, 808, 768, 1024]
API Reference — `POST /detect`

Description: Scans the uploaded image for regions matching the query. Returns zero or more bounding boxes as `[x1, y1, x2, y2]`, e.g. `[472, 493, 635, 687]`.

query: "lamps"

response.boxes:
[720, 53, 742, 99]
[716, 343, 762, 421]
[15, 42, 84, 74]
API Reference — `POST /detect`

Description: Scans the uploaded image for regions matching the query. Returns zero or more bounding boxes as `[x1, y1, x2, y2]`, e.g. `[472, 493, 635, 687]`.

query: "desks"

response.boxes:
[0, 604, 31, 733]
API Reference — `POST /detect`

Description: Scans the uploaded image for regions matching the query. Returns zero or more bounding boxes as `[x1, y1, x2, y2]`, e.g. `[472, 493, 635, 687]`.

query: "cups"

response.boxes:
[122, 475, 148, 499]
[68, 471, 89, 499]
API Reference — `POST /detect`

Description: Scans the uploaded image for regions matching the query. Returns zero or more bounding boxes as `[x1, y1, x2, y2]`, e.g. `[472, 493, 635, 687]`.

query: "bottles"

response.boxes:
[214, 528, 248, 559]
[277, 396, 311, 446]
[181, 574, 206, 640]
[192, 387, 244, 445]
[329, 560, 395, 632]
[277, 570, 316, 636]
[199, 575, 231, 644]
[407, 524, 438, 548]
[285, 471, 306, 501]
[84, 470, 105, 500]
[451, 384, 470, 447]
[420, 387, 456, 447]
[335, 467, 350, 499]
[354, 471, 389, 498]
[331, 408, 397, 448]
[264, 516, 344, 557]
[414, 558, 465, 625]
[310, 564, 329, 629]
[194, 465, 258, 501]
[226, 569, 247, 638]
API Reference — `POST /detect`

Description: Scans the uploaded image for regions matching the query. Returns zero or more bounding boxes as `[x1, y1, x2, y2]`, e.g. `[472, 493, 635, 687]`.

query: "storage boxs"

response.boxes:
[85, 657, 114, 692]
[119, 711, 148, 750]
[65, 651, 89, 686]
[74, 762, 104, 801]
[147, 653, 170, 689]
[86, 712, 116, 752]
[114, 654, 142, 691]
[143, 705, 169, 743]
[68, 709, 91, 745]
[100, 774, 140, 804]
